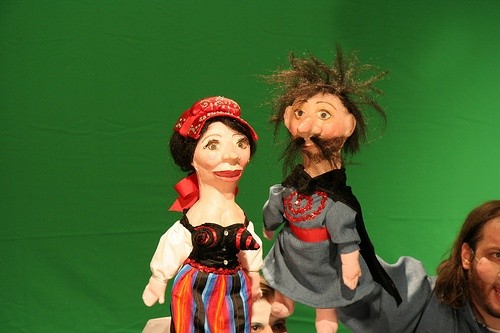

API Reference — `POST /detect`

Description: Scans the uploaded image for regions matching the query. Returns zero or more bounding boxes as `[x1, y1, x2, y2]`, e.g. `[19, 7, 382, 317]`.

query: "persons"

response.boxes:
[141, 95, 263, 333]
[244, 278, 289, 333]
[259, 42, 404, 333]
[334, 200, 500, 333]
